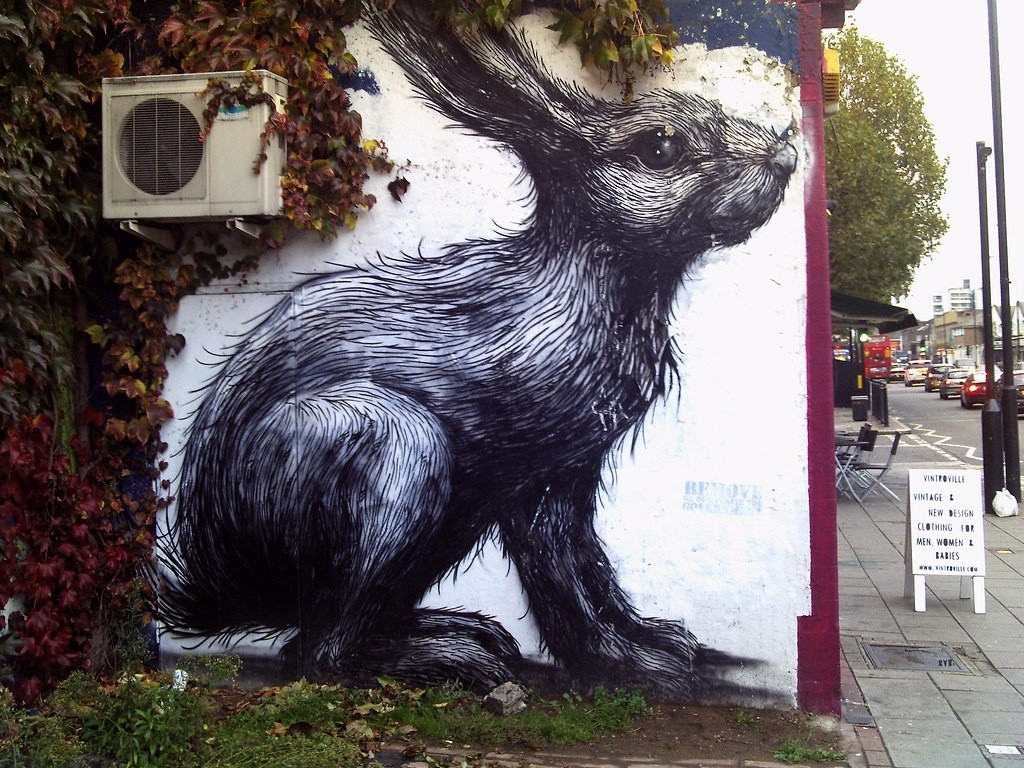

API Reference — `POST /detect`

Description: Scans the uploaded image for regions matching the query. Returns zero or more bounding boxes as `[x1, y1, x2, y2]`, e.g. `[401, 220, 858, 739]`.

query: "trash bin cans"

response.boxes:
[851, 396, 869, 422]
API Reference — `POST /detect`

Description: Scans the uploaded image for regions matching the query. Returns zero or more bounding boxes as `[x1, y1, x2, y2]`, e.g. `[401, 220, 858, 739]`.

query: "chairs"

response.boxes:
[834, 420, 905, 504]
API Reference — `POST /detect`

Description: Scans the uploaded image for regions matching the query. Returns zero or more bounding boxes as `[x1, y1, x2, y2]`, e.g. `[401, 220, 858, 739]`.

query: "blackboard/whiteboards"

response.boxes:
[903, 468, 986, 576]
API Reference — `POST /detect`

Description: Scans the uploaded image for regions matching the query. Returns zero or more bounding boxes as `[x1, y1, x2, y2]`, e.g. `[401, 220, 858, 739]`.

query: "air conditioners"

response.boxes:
[100, 68, 294, 250]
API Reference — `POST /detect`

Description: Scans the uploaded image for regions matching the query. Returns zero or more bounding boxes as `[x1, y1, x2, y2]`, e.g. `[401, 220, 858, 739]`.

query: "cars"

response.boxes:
[888, 344, 1024, 415]
